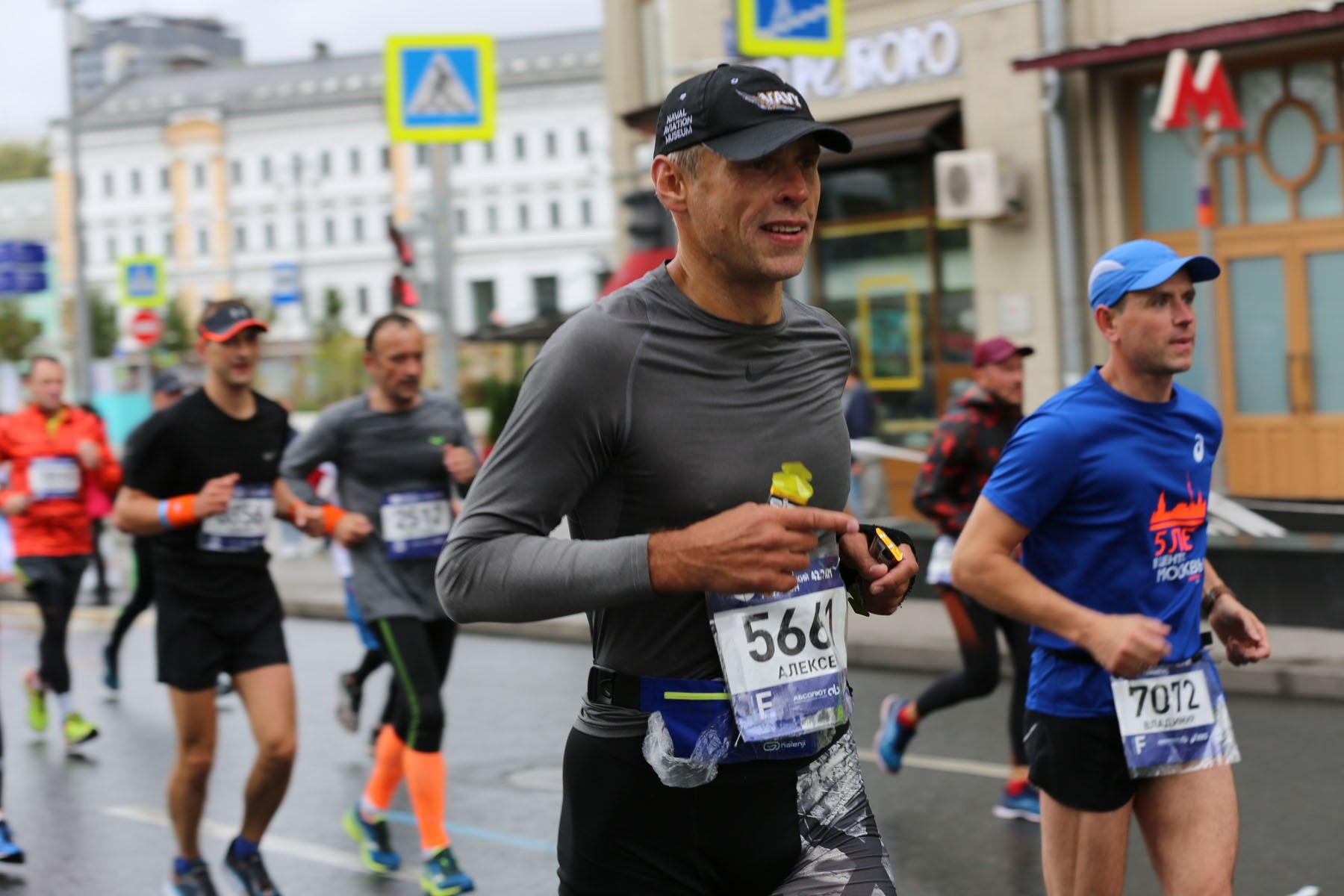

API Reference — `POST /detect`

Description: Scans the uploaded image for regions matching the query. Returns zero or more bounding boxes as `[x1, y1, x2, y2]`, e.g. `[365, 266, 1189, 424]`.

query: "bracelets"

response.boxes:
[164, 491, 196, 526]
[1195, 586, 1235, 620]
[285, 500, 307, 524]
[159, 500, 171, 531]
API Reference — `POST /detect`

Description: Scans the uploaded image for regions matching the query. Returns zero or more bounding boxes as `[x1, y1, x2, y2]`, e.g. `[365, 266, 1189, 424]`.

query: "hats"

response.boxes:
[651, 64, 855, 162]
[972, 339, 1036, 365]
[1087, 240, 1221, 312]
[152, 374, 182, 394]
[196, 305, 268, 349]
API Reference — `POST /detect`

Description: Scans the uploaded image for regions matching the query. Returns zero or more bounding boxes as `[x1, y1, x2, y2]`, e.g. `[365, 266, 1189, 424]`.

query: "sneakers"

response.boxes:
[63, 711, 98, 745]
[341, 799, 403, 874]
[24, 672, 48, 732]
[998, 782, 1042, 824]
[419, 847, 474, 896]
[872, 694, 919, 777]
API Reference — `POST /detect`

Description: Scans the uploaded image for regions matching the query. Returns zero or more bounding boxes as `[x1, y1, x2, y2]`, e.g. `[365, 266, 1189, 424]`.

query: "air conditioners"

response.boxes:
[933, 149, 1030, 224]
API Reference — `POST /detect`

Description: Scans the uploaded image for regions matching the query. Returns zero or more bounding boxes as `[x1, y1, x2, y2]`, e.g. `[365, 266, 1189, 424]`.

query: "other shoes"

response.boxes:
[341, 672, 359, 729]
[104, 645, 118, 690]
[0, 823, 24, 864]
[225, 837, 281, 896]
[170, 858, 219, 896]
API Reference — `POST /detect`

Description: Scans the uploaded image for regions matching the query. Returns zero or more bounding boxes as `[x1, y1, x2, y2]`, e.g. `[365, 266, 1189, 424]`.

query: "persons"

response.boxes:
[1, 293, 1041, 896]
[441, 63, 918, 896]
[951, 238, 1270, 896]
